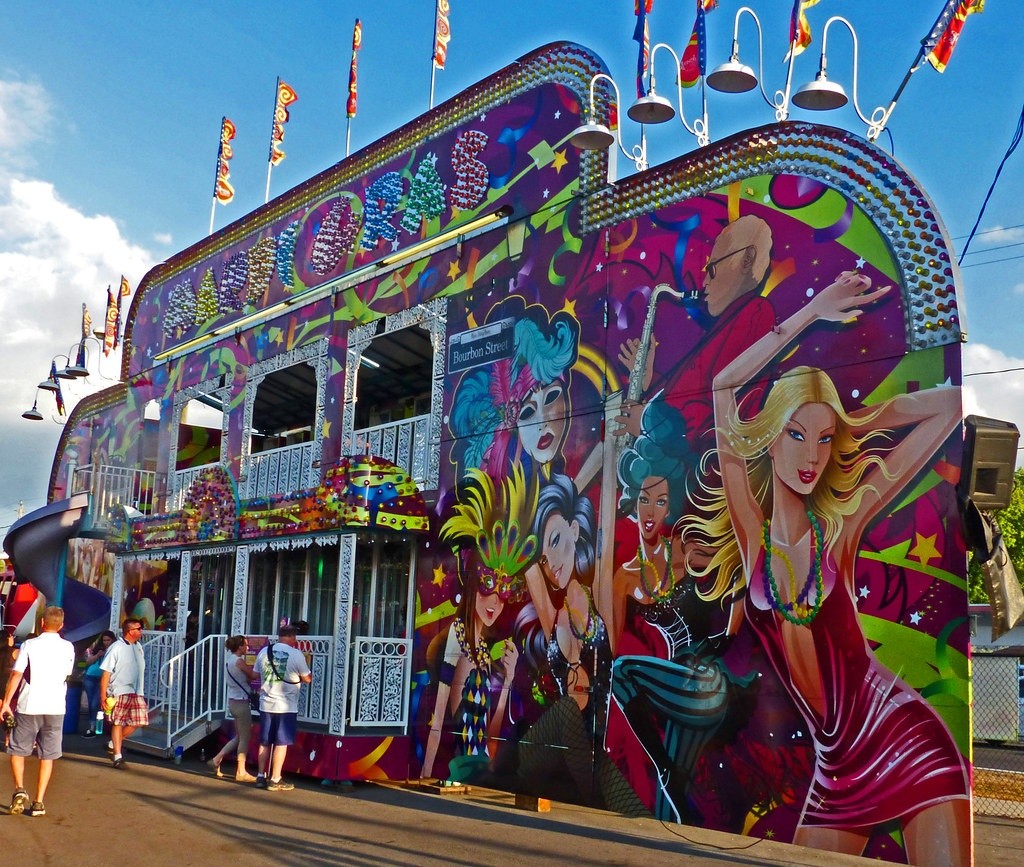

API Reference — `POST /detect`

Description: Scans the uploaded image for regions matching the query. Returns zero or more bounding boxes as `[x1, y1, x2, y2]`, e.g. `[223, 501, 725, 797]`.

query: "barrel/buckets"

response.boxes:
[63, 681, 82, 733]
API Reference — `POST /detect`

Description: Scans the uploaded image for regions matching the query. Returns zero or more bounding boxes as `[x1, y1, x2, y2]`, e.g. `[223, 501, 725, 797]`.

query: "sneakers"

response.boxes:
[256, 777, 265, 788]
[8, 788, 29, 814]
[104, 741, 115, 762]
[81, 730, 95, 739]
[113, 757, 129, 769]
[29, 800, 48, 816]
[267, 779, 294, 791]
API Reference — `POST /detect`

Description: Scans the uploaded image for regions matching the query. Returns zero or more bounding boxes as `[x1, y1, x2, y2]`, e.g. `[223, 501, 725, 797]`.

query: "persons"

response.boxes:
[0, 607, 76, 815]
[253, 625, 310, 791]
[80, 632, 115, 740]
[99, 618, 148, 766]
[0, 630, 38, 751]
[206, 636, 257, 783]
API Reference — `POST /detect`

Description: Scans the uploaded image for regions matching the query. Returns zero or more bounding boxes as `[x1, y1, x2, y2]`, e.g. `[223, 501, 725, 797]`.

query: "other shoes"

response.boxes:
[207, 759, 223, 777]
[236, 775, 256, 782]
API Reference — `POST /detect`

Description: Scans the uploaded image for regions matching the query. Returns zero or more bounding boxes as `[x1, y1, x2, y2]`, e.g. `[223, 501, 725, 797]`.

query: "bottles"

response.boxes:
[175, 746, 183, 765]
[200, 749, 205, 761]
[439, 780, 453, 786]
[95, 711, 104, 735]
[104, 695, 115, 715]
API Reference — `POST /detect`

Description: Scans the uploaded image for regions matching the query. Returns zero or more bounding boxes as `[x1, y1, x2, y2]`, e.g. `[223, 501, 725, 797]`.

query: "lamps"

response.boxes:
[791, 16, 886, 139]
[568, 73, 646, 173]
[66, 337, 120, 384]
[153, 205, 514, 362]
[19, 385, 73, 428]
[706, 7, 785, 121]
[627, 43, 708, 149]
[54, 343, 105, 397]
[37, 355, 91, 402]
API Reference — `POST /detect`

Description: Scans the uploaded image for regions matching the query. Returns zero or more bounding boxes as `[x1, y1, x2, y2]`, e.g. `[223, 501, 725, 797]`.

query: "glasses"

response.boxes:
[128, 627, 142, 631]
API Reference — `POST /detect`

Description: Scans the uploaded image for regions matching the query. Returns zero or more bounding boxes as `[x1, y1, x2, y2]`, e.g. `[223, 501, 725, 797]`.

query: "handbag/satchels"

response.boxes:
[248, 693, 260, 712]
